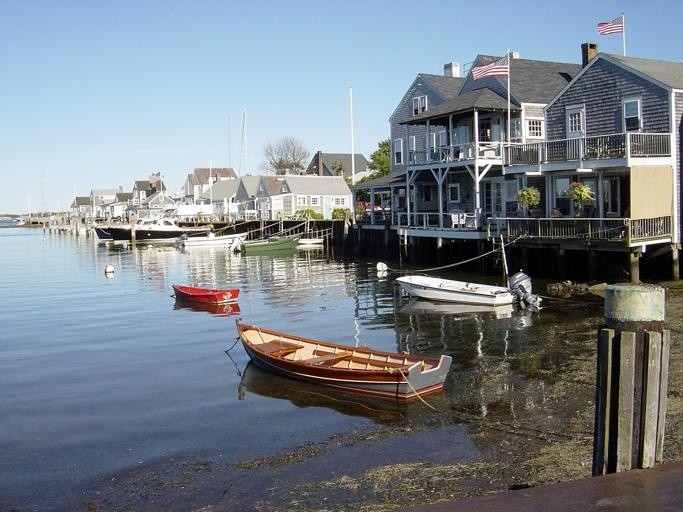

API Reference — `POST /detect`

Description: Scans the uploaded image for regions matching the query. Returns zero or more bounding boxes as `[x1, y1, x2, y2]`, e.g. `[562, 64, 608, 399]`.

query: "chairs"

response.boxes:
[448, 208, 466, 227]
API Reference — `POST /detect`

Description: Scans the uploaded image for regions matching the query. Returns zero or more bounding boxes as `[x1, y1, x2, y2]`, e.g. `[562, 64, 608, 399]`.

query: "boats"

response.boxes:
[395, 274, 518, 306]
[93, 223, 323, 257]
[173, 285, 239, 304]
[236, 321, 453, 404]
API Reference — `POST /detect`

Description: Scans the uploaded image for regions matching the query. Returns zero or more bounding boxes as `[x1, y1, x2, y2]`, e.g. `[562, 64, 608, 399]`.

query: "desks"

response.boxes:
[436, 147, 456, 163]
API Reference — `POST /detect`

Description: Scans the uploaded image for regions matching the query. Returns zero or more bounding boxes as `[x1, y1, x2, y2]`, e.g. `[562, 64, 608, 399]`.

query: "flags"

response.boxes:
[597, 16, 623, 35]
[471, 54, 509, 80]
